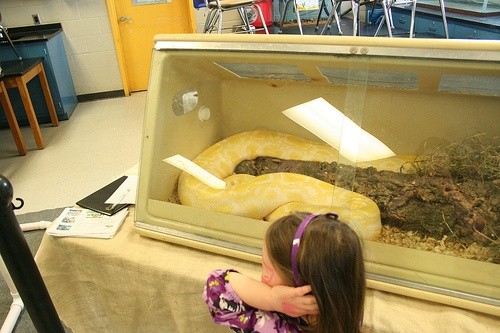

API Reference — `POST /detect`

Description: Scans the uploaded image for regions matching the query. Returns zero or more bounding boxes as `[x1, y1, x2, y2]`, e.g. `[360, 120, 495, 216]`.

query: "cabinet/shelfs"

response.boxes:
[1, 23, 77, 119]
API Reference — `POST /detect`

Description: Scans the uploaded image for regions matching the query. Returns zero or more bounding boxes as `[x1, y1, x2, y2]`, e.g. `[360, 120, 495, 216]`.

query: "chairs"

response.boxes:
[278, 0, 450, 39]
[193, 0, 267, 34]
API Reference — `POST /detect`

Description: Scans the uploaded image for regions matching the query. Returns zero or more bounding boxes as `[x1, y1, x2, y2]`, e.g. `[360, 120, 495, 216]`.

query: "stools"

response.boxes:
[0, 57, 59, 149]
[1, 73, 27, 156]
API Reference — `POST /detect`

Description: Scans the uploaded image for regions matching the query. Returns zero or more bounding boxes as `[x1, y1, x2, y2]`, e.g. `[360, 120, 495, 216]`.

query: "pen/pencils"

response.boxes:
[109, 189, 129, 211]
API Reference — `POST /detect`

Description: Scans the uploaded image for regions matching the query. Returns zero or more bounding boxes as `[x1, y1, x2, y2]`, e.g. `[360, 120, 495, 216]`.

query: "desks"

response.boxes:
[35, 184, 500, 333]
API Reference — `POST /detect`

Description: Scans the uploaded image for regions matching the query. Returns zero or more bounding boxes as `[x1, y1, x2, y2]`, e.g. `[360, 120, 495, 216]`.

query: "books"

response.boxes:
[74, 174, 131, 216]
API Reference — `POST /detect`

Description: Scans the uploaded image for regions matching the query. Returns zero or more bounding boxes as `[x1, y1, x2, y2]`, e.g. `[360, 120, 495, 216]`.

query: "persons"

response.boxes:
[203, 211, 366, 333]
